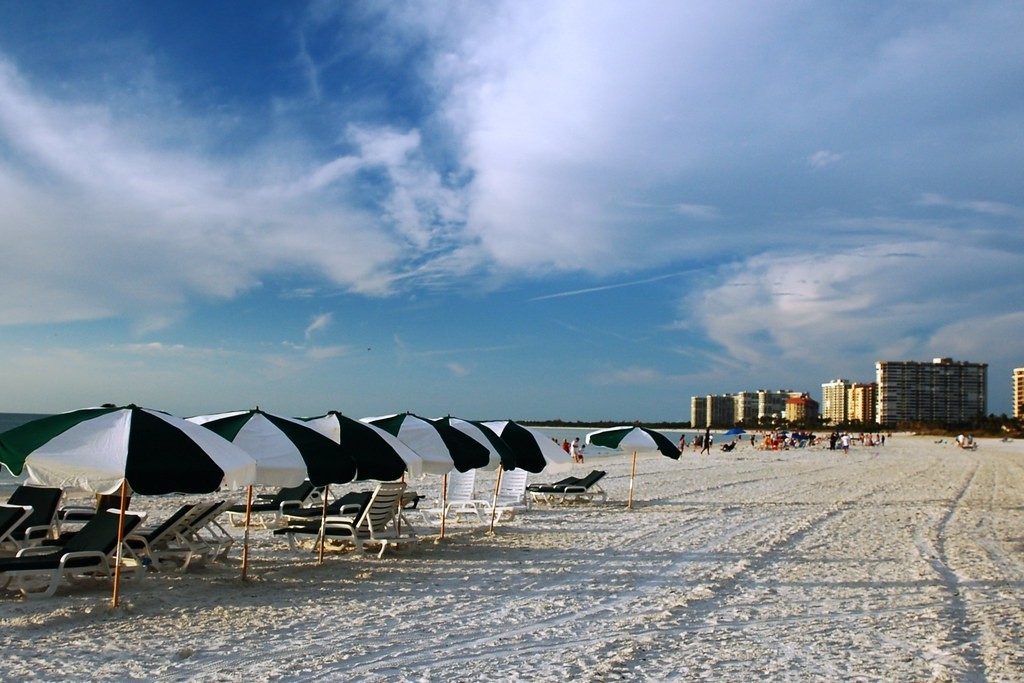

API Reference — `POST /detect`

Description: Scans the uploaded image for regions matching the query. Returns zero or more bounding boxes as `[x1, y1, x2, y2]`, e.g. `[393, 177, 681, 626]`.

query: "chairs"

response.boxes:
[486, 468, 528, 509]
[526, 469, 609, 507]
[434, 468, 480, 509]
[0, 478, 424, 600]
[420, 500, 528, 525]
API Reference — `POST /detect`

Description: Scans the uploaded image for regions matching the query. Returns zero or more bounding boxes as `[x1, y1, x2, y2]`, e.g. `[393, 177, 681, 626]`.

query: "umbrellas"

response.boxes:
[1, 403, 422, 609]
[779, 430, 789, 436]
[725, 429, 747, 435]
[586, 420, 682, 509]
[358, 414, 572, 551]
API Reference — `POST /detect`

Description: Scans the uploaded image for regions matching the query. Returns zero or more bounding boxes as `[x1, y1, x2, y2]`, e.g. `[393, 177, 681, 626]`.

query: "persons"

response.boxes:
[720, 440, 734, 452]
[955, 433, 977, 450]
[552, 436, 585, 464]
[749, 431, 813, 452]
[830, 431, 885, 454]
[678, 427, 714, 460]
